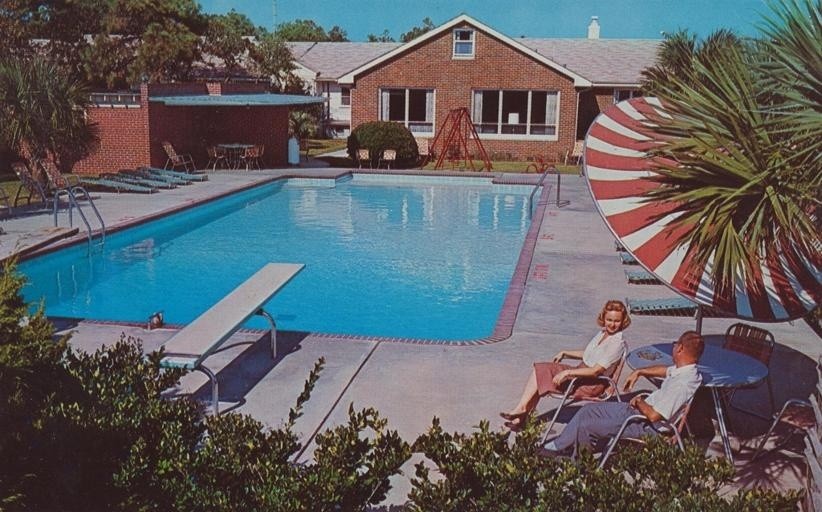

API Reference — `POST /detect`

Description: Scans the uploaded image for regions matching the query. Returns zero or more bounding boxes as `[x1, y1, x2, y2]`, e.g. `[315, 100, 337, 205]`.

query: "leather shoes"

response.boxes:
[504, 421, 521, 432]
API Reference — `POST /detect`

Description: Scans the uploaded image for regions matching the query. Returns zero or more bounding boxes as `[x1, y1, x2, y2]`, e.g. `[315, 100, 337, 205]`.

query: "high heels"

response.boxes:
[499, 411, 527, 427]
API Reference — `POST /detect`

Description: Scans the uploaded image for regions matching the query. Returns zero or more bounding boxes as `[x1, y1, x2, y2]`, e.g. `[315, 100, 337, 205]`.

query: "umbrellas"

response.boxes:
[580, 96, 821, 334]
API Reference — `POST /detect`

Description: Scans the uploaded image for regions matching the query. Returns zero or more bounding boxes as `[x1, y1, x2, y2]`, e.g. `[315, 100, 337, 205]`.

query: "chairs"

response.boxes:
[564, 140, 586, 168]
[0, 138, 267, 215]
[355, 138, 428, 171]
[541, 240, 822, 479]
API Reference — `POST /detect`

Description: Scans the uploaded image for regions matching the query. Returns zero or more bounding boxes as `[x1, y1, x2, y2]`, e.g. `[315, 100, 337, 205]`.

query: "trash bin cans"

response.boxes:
[288, 138, 300, 167]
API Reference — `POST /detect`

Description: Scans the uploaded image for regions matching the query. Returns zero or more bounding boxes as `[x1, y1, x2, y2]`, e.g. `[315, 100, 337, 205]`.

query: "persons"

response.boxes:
[543, 330, 704, 456]
[500, 301, 632, 427]
